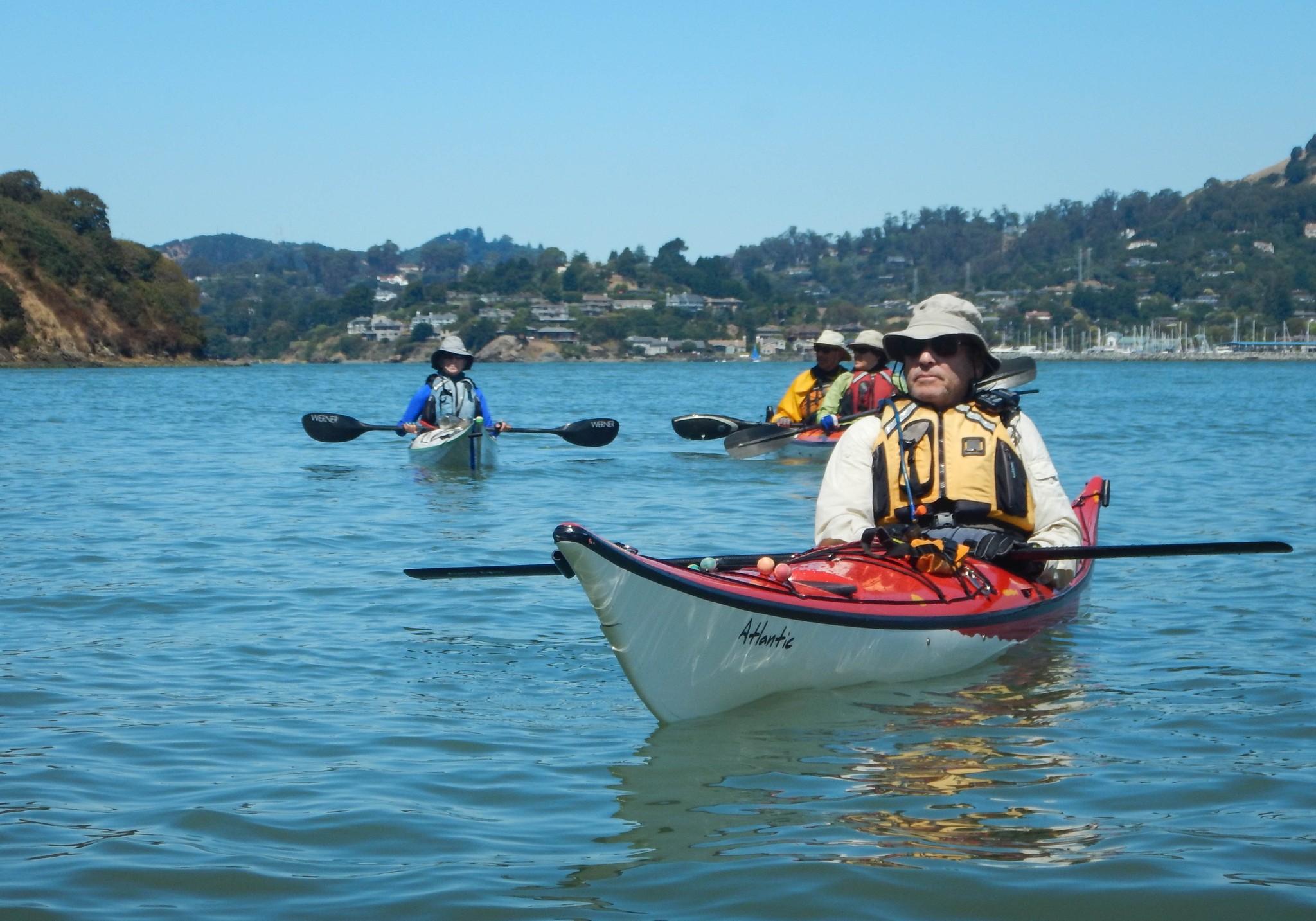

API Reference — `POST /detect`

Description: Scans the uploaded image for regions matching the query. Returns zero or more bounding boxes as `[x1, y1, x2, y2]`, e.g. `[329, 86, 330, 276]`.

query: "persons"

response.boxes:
[768, 329, 909, 433]
[393, 336, 511, 438]
[815, 293, 1083, 584]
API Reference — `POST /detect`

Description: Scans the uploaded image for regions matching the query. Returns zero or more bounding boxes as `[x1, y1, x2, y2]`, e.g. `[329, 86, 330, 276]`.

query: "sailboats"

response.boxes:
[751, 344, 762, 363]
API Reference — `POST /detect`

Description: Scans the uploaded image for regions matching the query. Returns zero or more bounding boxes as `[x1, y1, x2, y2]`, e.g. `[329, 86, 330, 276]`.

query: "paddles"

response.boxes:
[722, 356, 1037, 460]
[404, 540, 1296, 580]
[302, 411, 620, 448]
[671, 413, 806, 441]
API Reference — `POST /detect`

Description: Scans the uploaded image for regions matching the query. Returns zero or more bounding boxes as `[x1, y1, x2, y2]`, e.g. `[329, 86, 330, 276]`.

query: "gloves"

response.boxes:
[820, 414, 841, 430]
[881, 524, 910, 541]
[974, 533, 1045, 578]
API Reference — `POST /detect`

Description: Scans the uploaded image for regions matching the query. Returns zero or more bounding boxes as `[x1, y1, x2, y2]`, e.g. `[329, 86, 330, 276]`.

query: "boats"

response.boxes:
[550, 473, 1114, 726]
[403, 407, 503, 473]
[777, 426, 850, 461]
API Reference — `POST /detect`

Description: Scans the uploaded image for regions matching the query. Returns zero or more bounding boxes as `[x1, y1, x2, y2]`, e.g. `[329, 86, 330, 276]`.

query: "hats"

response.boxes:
[846, 330, 884, 349]
[882, 294, 1002, 382]
[806, 330, 852, 361]
[431, 336, 474, 371]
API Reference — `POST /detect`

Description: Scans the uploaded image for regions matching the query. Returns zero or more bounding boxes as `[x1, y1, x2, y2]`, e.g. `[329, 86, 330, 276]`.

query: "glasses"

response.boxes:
[902, 339, 979, 357]
[444, 353, 465, 360]
[854, 348, 871, 354]
[814, 345, 838, 354]
